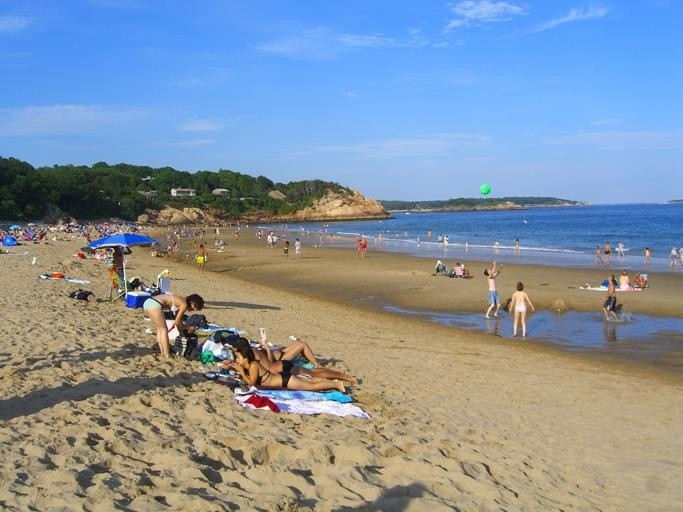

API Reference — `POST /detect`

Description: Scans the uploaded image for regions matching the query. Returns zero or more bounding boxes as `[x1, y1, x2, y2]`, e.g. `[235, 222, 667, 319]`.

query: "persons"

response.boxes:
[483, 261, 504, 320]
[163, 221, 239, 271]
[677, 248, 683, 262]
[600, 275, 619, 321]
[112, 245, 132, 295]
[233, 343, 346, 395]
[594, 245, 602, 265]
[142, 293, 205, 358]
[668, 246, 679, 267]
[221, 337, 360, 385]
[507, 281, 535, 338]
[603, 241, 612, 265]
[634, 273, 646, 291]
[642, 246, 652, 266]
[1, 220, 136, 244]
[615, 241, 625, 257]
[414, 229, 521, 288]
[232, 335, 325, 369]
[618, 269, 634, 290]
[255, 223, 335, 257]
[354, 231, 384, 257]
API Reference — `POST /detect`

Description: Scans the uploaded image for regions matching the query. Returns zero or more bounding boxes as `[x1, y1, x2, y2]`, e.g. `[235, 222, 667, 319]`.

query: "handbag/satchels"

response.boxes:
[70, 291, 94, 301]
[175, 330, 198, 357]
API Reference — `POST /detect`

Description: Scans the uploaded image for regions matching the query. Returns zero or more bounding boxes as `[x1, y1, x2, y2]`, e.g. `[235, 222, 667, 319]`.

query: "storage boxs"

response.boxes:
[126, 291, 150, 307]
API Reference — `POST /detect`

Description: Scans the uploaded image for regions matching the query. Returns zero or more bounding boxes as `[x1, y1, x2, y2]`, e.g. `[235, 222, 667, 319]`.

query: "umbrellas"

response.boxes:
[79, 230, 157, 299]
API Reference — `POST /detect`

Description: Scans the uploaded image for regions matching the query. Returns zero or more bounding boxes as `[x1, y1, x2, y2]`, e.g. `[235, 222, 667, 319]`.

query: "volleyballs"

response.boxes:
[478, 183, 490, 194]
